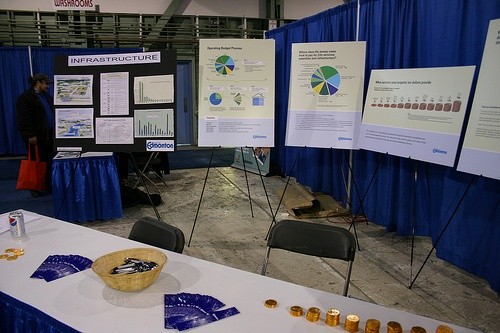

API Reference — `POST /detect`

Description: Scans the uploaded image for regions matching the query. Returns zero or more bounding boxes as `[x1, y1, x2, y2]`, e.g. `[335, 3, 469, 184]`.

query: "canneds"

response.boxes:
[8, 211, 25, 238]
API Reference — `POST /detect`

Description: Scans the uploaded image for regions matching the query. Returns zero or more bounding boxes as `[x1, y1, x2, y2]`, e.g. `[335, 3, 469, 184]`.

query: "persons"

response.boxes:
[15, 72, 56, 196]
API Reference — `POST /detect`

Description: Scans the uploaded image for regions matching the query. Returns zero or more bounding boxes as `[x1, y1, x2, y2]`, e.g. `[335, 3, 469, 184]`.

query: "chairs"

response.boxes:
[127, 216, 185, 254]
[262, 219, 356, 296]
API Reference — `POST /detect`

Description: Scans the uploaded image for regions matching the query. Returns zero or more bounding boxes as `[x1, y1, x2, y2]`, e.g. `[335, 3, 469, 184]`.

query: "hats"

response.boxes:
[33, 73, 53, 83]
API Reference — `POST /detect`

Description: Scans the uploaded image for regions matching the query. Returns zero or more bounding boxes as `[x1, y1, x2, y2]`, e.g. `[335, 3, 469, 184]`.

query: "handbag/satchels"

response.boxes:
[15, 142, 47, 191]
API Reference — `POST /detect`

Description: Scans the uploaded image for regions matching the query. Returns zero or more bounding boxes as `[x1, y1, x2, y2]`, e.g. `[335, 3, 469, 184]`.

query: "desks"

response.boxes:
[0, 205, 488, 333]
[51, 150, 123, 223]
